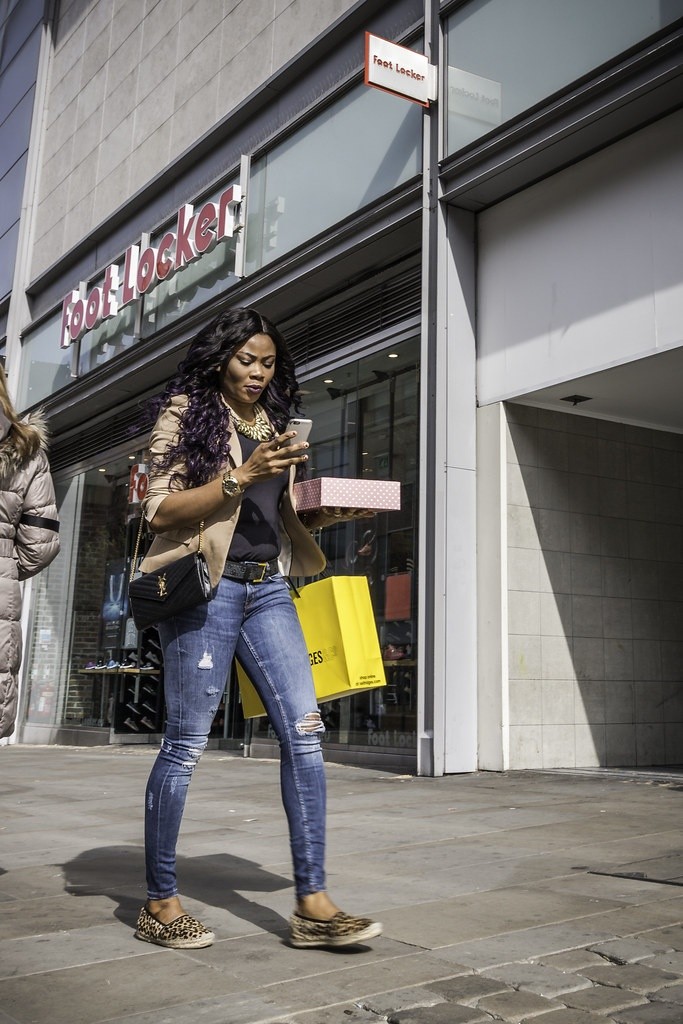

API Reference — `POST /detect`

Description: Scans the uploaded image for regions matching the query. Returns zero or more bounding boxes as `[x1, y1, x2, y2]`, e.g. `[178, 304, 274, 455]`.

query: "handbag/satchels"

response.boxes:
[235, 575, 387, 719]
[127, 503, 211, 631]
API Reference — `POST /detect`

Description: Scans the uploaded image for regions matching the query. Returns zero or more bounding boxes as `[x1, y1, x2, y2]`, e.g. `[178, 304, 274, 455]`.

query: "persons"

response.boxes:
[133, 306, 386, 951]
[0, 354, 68, 738]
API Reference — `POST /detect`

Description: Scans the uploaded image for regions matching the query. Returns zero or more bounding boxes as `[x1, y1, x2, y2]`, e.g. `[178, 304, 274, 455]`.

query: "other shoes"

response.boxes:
[286, 910, 383, 947]
[134, 904, 215, 948]
[384, 645, 410, 660]
[85, 637, 162, 731]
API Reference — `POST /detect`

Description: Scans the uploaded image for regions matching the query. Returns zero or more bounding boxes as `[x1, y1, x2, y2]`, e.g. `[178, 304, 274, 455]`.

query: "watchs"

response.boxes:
[222, 470, 245, 498]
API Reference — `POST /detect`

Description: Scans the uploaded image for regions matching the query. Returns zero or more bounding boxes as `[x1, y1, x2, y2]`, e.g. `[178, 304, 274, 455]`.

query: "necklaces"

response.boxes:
[230, 403, 274, 442]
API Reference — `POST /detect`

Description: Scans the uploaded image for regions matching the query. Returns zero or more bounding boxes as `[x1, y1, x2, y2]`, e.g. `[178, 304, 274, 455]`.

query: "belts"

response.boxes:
[222, 560, 279, 582]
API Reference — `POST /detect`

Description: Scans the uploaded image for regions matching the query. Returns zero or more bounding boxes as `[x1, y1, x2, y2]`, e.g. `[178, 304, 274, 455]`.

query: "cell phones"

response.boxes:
[278, 419, 313, 459]
